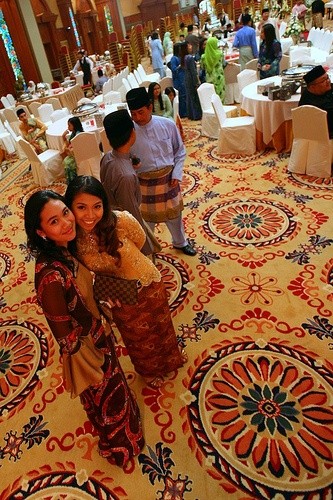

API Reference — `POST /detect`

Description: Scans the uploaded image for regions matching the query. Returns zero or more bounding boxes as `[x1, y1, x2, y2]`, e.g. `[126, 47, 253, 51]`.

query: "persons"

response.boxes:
[96, 62, 116, 97]
[312, 0, 326, 29]
[234, 15, 258, 71]
[170, 19, 228, 121]
[65, 174, 188, 388]
[257, 24, 282, 80]
[15, 76, 36, 96]
[147, 82, 173, 118]
[99, 109, 162, 265]
[72, 51, 95, 97]
[164, 87, 176, 107]
[126, 87, 196, 256]
[217, 7, 250, 38]
[297, 65, 333, 140]
[145, 31, 173, 79]
[24, 189, 146, 468]
[258, 9, 281, 41]
[17, 109, 48, 155]
[292, 0, 308, 27]
[60, 116, 83, 185]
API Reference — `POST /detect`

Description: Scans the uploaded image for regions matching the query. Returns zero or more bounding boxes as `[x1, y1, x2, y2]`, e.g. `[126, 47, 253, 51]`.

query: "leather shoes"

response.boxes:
[173, 245, 197, 256]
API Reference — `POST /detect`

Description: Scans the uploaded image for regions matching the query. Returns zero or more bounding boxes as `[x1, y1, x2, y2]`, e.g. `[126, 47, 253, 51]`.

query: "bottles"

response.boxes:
[132, 154, 140, 166]
[23, 80, 28, 91]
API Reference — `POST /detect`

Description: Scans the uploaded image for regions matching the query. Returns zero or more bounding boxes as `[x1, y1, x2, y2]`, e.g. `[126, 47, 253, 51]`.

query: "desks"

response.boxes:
[65, 64, 113, 86]
[325, 2, 333, 20]
[47, 102, 128, 152]
[16, 84, 85, 111]
[220, 38, 260, 62]
[240, 68, 333, 152]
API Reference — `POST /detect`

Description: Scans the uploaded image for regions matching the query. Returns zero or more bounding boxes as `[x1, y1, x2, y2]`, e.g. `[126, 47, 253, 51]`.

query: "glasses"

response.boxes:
[313, 75, 330, 86]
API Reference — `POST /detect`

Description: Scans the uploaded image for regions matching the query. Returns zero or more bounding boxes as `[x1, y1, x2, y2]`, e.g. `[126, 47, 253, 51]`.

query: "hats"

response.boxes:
[103, 109, 133, 136]
[302, 65, 325, 84]
[126, 87, 149, 110]
[262, 9, 269, 13]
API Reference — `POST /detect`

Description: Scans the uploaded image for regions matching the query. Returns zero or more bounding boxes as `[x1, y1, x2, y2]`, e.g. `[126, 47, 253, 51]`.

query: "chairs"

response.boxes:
[288, 105, 333, 178]
[197, 83, 238, 140]
[223, 62, 240, 105]
[199, 0, 304, 27]
[211, 94, 257, 153]
[236, 68, 258, 91]
[280, 26, 333, 74]
[0, 12, 195, 184]
[245, 58, 260, 76]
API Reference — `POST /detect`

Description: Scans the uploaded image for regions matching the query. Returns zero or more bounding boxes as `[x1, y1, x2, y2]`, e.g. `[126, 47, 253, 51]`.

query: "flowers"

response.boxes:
[271, 3, 281, 12]
[281, 18, 305, 38]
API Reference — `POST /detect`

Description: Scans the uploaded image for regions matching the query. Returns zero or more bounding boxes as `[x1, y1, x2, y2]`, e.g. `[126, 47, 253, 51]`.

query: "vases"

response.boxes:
[291, 34, 298, 44]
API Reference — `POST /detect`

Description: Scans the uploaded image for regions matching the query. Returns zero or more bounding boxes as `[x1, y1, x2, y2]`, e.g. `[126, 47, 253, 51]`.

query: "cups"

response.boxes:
[91, 120, 94, 125]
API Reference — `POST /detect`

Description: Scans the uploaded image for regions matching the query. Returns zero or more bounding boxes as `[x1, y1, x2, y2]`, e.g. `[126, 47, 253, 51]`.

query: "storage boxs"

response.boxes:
[259, 77, 306, 100]
[118, 104, 127, 111]
[94, 112, 105, 128]
